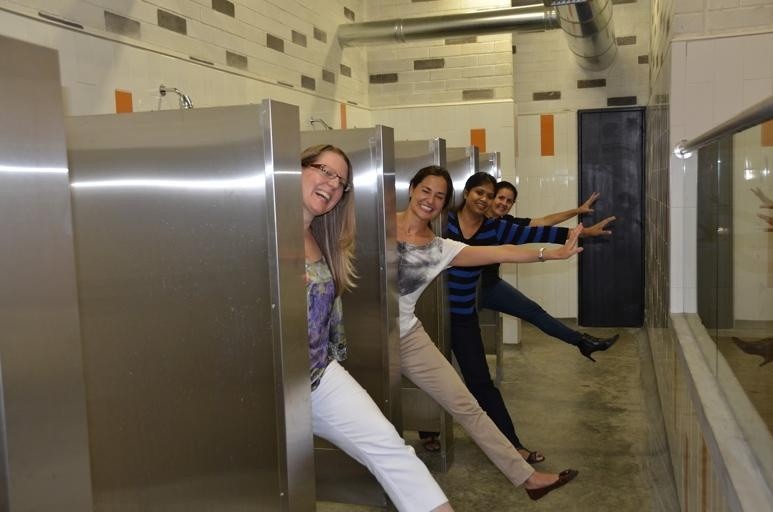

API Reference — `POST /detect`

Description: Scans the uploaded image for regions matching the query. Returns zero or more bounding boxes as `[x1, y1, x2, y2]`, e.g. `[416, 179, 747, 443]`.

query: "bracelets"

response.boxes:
[538, 246, 545, 264]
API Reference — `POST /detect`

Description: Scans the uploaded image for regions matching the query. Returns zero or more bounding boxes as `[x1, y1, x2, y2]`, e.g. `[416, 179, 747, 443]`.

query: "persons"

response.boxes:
[755, 212, 773, 231]
[749, 185, 773, 209]
[478, 181, 621, 364]
[301, 142, 457, 512]
[395, 164, 585, 502]
[417, 172, 618, 464]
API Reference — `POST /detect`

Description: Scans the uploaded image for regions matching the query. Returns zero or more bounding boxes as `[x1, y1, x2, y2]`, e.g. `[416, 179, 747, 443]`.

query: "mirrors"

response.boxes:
[696, 119, 773, 496]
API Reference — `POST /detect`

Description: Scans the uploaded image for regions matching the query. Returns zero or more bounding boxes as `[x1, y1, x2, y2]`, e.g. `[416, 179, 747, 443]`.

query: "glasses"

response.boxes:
[310, 164, 351, 192]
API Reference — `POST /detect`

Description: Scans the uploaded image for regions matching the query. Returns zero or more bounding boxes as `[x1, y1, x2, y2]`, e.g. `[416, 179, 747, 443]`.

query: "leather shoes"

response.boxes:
[525, 469, 578, 500]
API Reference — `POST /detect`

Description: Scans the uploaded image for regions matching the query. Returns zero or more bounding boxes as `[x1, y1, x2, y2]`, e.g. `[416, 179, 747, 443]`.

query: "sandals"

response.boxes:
[425, 435, 440, 450]
[518, 449, 545, 463]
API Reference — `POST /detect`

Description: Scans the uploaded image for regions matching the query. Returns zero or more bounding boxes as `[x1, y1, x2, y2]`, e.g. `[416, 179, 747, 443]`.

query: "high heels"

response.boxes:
[576, 333, 620, 363]
